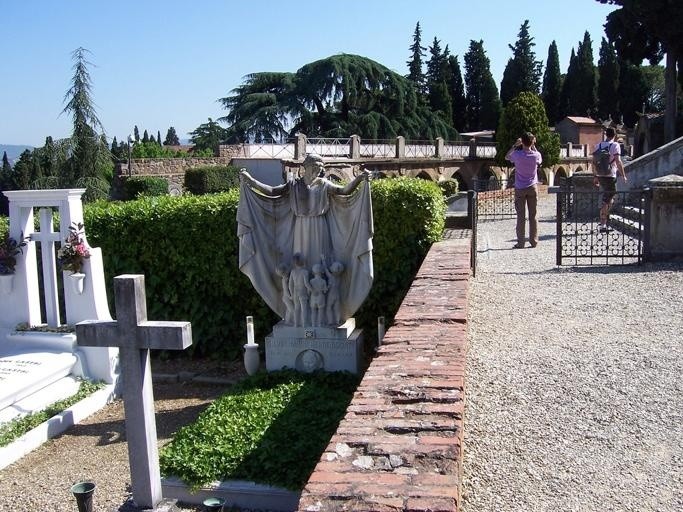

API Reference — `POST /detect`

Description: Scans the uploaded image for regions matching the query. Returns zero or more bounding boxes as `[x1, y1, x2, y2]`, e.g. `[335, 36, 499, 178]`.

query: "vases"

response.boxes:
[0, 275, 13, 294]
[68, 273, 85, 295]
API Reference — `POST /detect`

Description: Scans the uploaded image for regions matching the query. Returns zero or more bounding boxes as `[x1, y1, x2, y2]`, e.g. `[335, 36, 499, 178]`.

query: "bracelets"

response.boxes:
[594, 175, 597, 177]
[512, 144, 517, 148]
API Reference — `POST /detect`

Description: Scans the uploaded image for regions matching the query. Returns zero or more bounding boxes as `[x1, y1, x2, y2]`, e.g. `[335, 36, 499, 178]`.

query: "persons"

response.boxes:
[235, 153, 376, 330]
[591, 127, 628, 233]
[288, 251, 317, 330]
[306, 264, 330, 328]
[320, 253, 346, 326]
[504, 131, 544, 248]
[274, 262, 294, 326]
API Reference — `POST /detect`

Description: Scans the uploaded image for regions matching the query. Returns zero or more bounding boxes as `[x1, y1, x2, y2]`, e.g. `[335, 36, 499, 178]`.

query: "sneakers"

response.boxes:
[600, 226, 615, 234]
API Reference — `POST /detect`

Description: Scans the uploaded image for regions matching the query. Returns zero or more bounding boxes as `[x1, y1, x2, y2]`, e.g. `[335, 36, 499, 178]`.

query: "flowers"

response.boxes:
[0, 230, 31, 275]
[56, 222, 91, 274]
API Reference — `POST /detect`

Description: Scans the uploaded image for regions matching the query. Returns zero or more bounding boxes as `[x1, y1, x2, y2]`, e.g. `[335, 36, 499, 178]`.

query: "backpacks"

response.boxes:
[593, 142, 617, 178]
